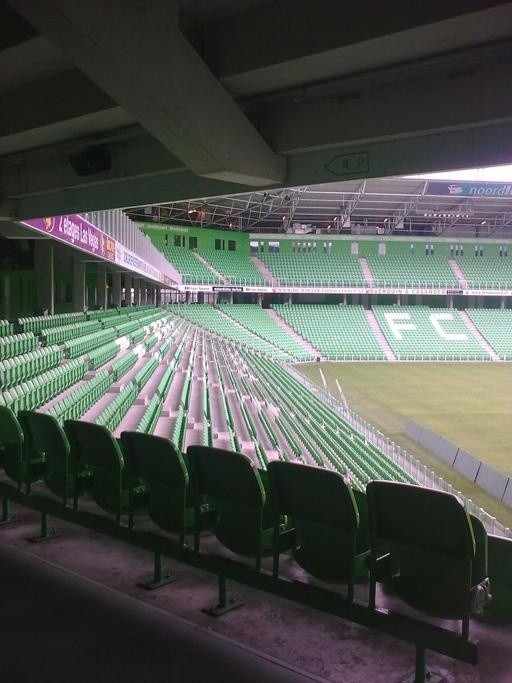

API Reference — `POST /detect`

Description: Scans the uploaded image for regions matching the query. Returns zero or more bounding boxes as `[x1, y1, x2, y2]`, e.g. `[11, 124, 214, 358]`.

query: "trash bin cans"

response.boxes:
[93, 304, 104, 310]
[121, 303, 129, 307]
[132, 303, 137, 306]
[32, 304, 49, 317]
[111, 304, 119, 308]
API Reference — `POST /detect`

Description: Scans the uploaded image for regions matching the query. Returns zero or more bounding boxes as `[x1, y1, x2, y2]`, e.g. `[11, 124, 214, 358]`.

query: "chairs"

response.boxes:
[0, 220, 512, 683]
